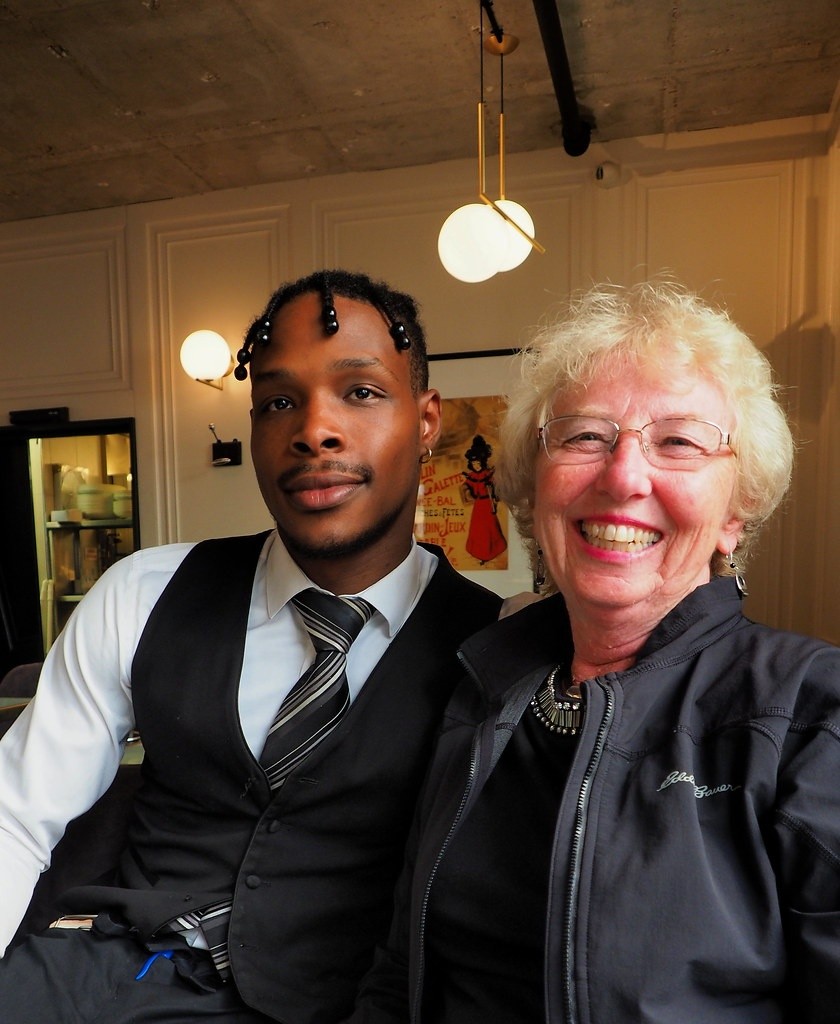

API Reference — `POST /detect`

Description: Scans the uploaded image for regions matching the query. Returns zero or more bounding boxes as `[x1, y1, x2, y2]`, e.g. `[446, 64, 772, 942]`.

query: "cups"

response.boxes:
[80, 559, 98, 594]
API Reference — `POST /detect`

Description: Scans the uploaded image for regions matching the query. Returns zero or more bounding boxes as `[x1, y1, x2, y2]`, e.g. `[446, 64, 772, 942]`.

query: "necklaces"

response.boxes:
[530, 665, 587, 734]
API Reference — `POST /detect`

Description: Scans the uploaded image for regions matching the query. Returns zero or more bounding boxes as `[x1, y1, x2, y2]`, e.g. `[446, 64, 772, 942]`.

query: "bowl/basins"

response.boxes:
[111, 491, 132, 518]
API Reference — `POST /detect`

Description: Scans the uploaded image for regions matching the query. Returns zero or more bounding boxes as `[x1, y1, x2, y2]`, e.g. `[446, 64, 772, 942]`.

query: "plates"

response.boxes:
[76, 484, 126, 519]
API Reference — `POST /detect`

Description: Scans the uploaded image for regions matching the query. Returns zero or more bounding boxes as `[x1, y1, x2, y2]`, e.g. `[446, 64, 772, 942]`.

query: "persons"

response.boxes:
[0, 269, 548, 1024]
[385, 281, 840, 1024]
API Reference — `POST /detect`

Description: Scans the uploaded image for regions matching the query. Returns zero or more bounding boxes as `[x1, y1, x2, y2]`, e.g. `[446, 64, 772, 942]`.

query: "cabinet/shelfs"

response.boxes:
[0, 418, 141, 671]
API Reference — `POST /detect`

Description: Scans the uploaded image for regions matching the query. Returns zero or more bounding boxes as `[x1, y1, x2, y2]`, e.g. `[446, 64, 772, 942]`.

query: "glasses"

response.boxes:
[537, 416, 738, 471]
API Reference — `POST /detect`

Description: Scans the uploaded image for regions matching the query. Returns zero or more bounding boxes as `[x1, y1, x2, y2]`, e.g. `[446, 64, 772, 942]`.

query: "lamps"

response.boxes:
[180, 330, 235, 392]
[437, 0, 546, 284]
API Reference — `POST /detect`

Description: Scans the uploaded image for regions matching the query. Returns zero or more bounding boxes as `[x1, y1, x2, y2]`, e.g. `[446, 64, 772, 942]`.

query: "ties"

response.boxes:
[156, 587, 378, 984]
